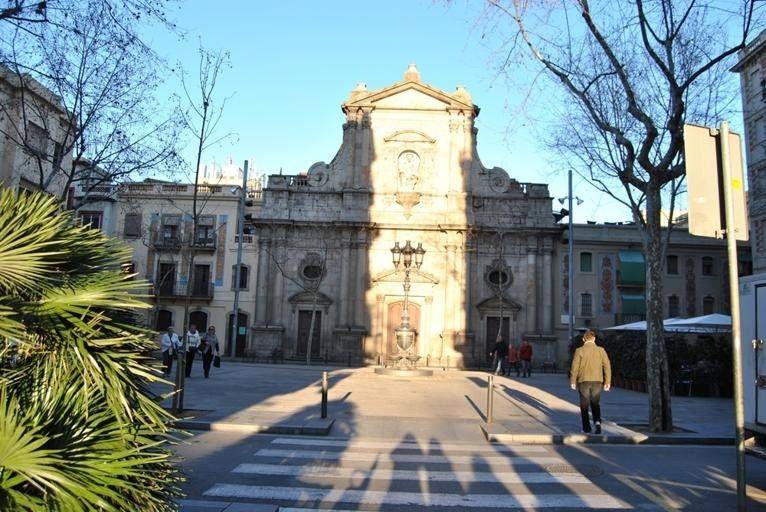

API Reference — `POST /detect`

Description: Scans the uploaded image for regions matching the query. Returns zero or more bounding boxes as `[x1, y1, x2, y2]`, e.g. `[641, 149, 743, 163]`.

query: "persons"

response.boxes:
[491, 335, 507, 376]
[567, 330, 613, 435]
[186, 325, 202, 376]
[507, 344, 520, 377]
[200, 326, 219, 380]
[161, 326, 179, 377]
[519, 339, 533, 377]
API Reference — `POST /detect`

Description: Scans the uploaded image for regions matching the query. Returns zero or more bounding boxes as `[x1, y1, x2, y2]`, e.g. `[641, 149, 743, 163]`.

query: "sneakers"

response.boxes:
[580, 421, 602, 435]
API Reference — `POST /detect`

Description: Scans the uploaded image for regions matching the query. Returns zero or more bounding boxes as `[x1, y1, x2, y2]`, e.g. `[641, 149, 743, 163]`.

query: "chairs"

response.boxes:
[242, 345, 284, 364]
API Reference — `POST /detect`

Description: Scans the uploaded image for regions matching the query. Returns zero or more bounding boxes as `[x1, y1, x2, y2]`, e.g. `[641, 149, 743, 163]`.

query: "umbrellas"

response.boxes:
[665, 312, 733, 336]
[604, 316, 686, 330]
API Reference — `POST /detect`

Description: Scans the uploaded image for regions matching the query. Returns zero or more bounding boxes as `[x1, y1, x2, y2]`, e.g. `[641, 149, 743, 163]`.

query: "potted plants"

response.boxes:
[599, 331, 648, 394]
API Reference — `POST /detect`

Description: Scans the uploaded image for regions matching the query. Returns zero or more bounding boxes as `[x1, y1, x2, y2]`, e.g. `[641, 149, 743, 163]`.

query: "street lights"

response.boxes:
[390, 240, 426, 379]
[230, 159, 253, 359]
[552, 170, 584, 369]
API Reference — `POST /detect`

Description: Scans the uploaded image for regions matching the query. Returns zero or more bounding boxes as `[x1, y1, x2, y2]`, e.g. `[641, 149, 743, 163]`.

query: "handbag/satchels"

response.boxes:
[213, 356, 220, 368]
[514, 361, 523, 371]
